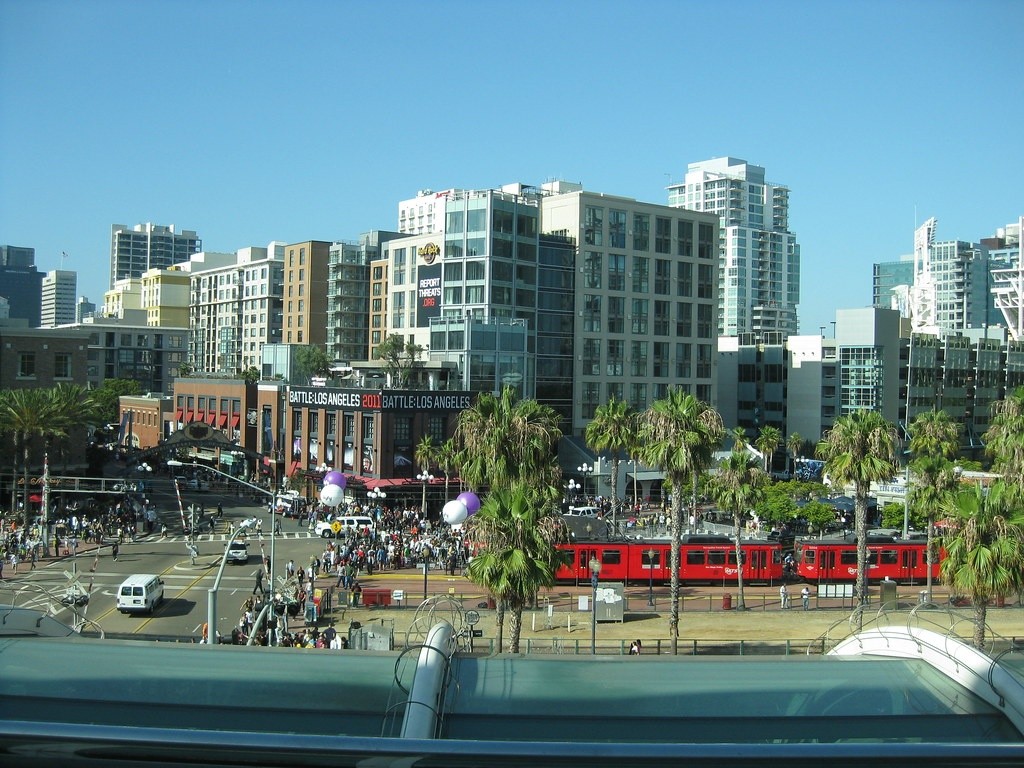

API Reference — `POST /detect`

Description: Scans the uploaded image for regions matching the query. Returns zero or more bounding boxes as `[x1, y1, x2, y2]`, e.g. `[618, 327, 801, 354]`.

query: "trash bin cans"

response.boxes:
[723, 593, 732, 609]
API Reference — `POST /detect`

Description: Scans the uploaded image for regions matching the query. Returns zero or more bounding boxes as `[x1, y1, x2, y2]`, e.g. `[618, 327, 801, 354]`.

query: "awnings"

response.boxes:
[218, 448, 240, 465]
[627, 470, 720, 485]
[376, 476, 464, 491]
[286, 460, 376, 489]
[188, 448, 213, 461]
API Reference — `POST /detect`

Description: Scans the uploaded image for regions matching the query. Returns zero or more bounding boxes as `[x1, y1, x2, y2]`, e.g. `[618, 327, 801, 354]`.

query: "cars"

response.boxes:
[187, 479, 206, 485]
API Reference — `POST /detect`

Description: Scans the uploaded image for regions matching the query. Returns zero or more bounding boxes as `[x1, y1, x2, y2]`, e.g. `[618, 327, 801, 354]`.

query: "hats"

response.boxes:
[355, 581, 359, 584]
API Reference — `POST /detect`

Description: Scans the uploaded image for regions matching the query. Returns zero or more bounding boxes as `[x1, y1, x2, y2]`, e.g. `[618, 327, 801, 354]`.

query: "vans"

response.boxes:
[116, 574, 164, 615]
[562, 506, 601, 519]
[268, 494, 308, 519]
[173, 476, 187, 483]
[315, 516, 373, 538]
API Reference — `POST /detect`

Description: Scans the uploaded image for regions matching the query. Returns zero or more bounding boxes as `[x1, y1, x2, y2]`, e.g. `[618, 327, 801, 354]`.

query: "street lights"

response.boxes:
[421, 545, 430, 605]
[167, 461, 275, 647]
[646, 547, 655, 606]
[863, 546, 872, 595]
[104, 423, 114, 452]
[416, 470, 434, 524]
[796, 455, 809, 485]
[367, 487, 386, 518]
[902, 450, 911, 541]
[562, 478, 581, 507]
[307, 554, 317, 627]
[588, 554, 602, 656]
[138, 463, 153, 492]
[577, 463, 593, 500]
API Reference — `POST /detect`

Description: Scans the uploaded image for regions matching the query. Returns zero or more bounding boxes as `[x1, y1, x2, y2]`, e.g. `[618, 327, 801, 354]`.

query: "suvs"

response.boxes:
[224, 540, 250, 564]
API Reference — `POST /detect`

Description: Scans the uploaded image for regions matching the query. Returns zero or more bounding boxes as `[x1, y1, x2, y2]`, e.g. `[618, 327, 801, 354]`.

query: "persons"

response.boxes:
[201, 494, 848, 650]
[207, 514, 216, 536]
[274, 518, 281, 536]
[0, 492, 157, 575]
[111, 541, 119, 562]
[252, 568, 266, 594]
[160, 524, 169, 538]
[150, 456, 271, 502]
[217, 500, 223, 519]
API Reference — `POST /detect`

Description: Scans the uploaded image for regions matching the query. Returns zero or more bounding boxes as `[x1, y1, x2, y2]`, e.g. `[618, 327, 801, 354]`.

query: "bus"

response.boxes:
[793, 532, 951, 585]
[552, 533, 782, 586]
